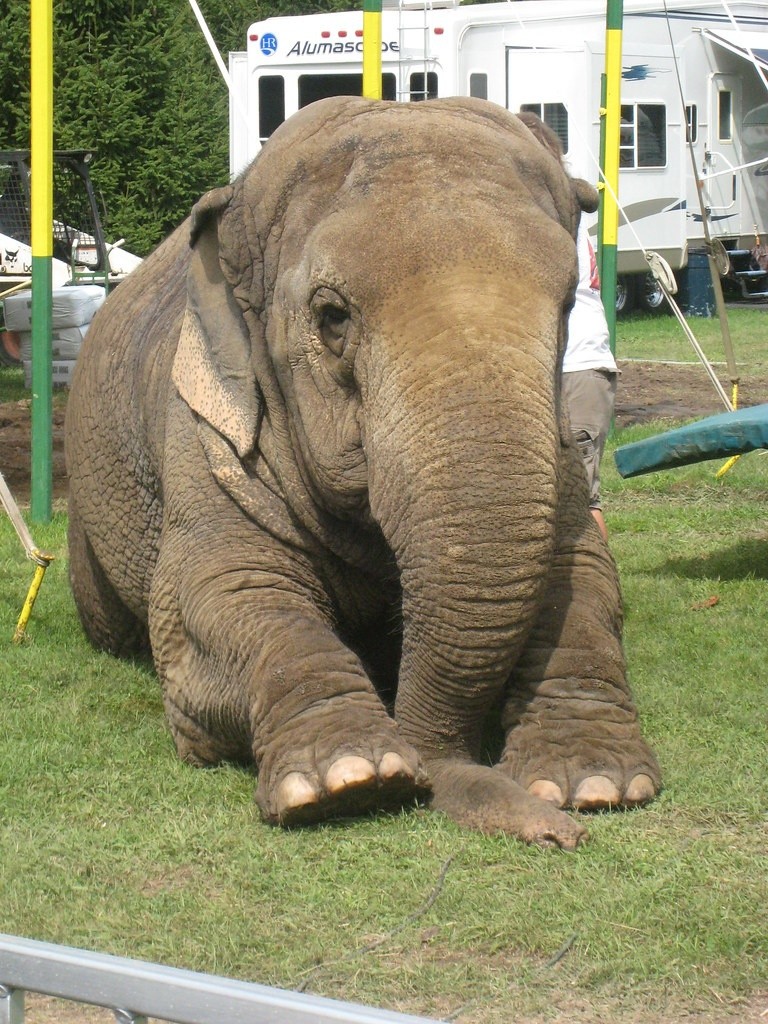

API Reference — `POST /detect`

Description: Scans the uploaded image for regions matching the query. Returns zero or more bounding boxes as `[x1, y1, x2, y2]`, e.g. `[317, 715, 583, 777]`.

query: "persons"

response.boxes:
[562, 225, 623, 544]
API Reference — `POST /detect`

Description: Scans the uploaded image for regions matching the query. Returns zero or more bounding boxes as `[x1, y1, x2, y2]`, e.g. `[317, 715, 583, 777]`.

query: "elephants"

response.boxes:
[62, 94, 663, 844]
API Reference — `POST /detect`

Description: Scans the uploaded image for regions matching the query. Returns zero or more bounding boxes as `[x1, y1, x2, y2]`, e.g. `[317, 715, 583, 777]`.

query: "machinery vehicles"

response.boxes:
[1, 148, 146, 368]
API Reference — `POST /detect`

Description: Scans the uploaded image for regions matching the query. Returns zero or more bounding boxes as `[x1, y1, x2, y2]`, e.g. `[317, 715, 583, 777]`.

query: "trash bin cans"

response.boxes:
[675, 247, 720, 319]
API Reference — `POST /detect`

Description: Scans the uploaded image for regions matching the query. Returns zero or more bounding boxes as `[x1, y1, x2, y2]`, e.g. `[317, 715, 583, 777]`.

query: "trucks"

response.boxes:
[226, 0, 768, 321]
